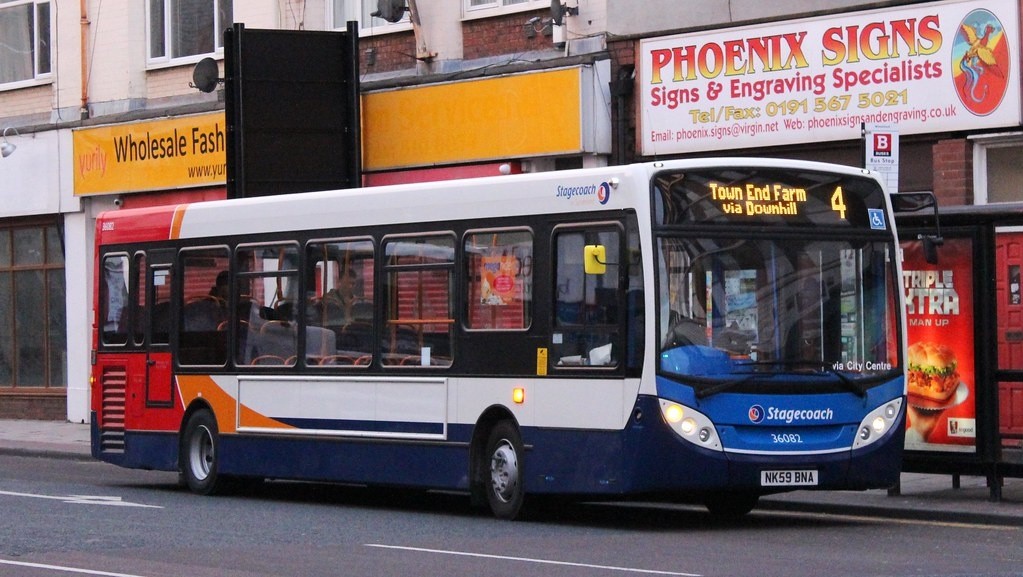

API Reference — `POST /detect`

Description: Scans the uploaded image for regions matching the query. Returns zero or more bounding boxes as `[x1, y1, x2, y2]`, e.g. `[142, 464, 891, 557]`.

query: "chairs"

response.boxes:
[114, 292, 430, 367]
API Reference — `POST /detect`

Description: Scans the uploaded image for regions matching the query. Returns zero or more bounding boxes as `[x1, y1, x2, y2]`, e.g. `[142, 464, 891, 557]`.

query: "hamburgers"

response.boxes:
[906, 341, 961, 401]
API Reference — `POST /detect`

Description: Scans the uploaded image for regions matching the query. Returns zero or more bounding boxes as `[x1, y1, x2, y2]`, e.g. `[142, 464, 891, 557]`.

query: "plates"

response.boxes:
[909, 381, 968, 411]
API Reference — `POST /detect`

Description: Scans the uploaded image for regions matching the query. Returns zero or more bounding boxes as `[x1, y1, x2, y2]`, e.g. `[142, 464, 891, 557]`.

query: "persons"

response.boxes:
[320, 266, 367, 317]
[208, 271, 230, 299]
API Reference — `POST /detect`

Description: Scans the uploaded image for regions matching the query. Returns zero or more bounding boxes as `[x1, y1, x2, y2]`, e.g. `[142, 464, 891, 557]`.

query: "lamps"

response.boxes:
[0, 126, 35, 157]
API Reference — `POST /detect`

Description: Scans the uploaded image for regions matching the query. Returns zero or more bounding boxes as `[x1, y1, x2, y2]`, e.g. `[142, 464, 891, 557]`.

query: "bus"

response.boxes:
[91, 157, 907, 523]
